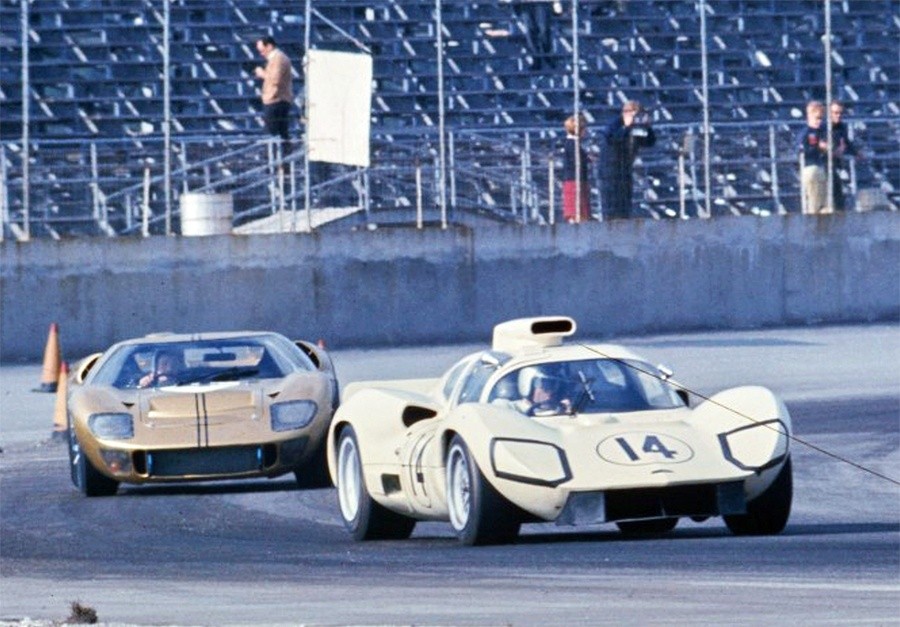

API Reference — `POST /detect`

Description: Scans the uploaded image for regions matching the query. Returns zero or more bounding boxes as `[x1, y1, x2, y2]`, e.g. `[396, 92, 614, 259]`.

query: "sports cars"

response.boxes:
[67, 333, 347, 488]
[326, 315, 797, 546]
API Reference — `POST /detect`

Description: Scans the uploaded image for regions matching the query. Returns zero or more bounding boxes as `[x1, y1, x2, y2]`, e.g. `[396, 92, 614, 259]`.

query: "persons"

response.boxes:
[135, 348, 185, 389]
[489, 367, 581, 415]
[251, 37, 293, 176]
[798, 98, 853, 214]
[560, 113, 596, 223]
[597, 100, 655, 222]
[508, 1, 556, 70]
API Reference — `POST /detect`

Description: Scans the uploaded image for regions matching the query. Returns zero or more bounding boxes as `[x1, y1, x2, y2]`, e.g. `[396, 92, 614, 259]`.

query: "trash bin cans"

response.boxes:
[179, 193, 234, 236]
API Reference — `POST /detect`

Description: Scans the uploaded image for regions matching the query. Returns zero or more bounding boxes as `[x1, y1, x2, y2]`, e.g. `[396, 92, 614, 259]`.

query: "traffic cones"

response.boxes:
[30, 323, 62, 391]
[47, 360, 73, 447]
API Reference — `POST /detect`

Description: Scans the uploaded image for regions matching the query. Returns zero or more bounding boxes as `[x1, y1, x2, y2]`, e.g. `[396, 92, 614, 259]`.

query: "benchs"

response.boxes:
[0, 1, 897, 240]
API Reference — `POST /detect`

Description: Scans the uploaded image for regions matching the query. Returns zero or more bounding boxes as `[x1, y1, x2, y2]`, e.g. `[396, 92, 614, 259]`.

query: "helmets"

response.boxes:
[518, 366, 568, 398]
